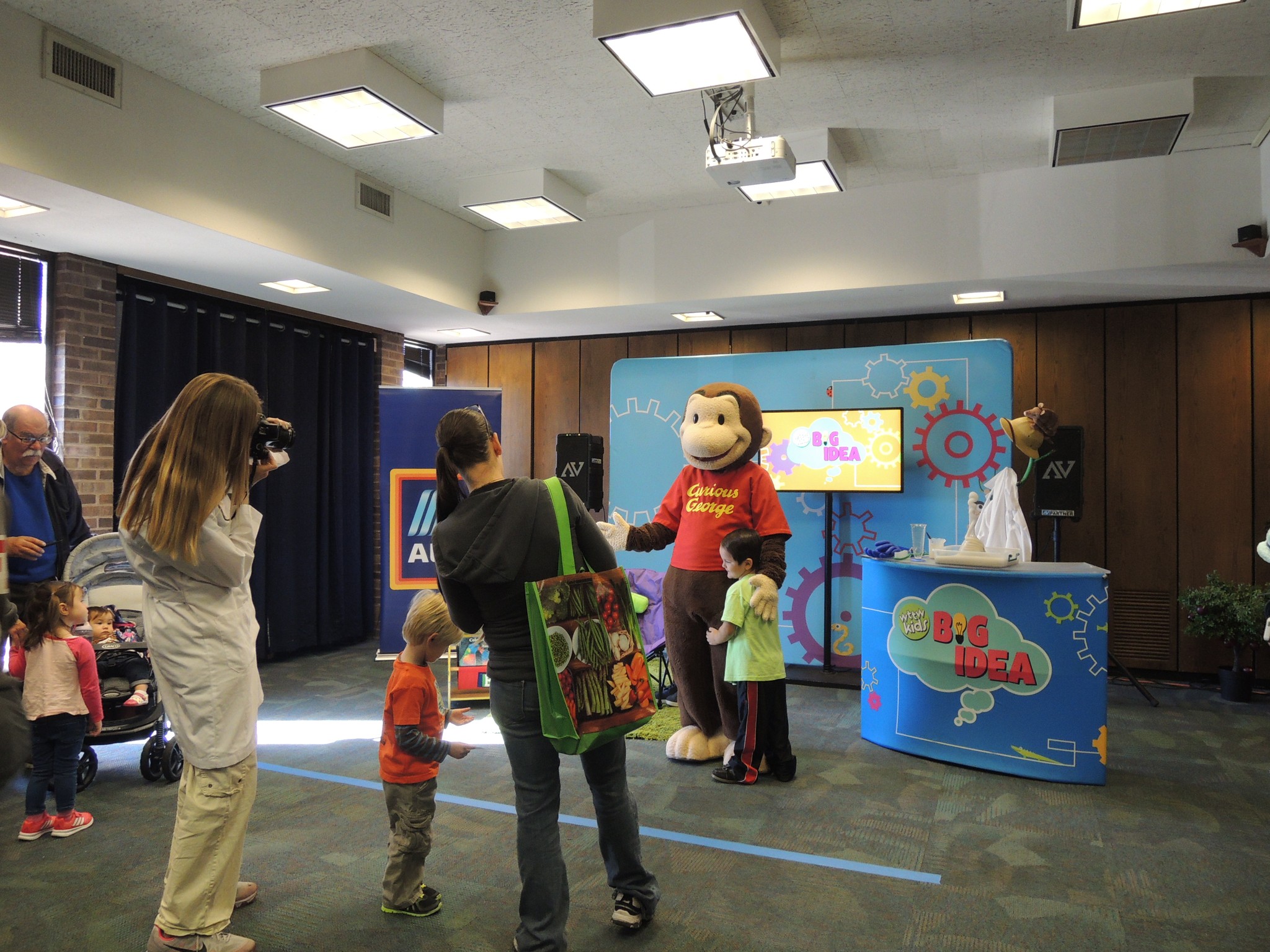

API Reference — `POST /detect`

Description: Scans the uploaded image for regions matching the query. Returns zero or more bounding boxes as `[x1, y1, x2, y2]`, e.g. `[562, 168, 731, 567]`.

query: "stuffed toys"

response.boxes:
[596, 382, 793, 765]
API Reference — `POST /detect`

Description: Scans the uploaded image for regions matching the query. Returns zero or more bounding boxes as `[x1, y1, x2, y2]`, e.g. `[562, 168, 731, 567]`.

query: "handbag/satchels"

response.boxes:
[523, 476, 659, 756]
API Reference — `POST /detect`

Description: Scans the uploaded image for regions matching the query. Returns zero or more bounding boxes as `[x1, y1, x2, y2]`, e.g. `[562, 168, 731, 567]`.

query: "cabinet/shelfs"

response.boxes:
[448, 634, 490, 709]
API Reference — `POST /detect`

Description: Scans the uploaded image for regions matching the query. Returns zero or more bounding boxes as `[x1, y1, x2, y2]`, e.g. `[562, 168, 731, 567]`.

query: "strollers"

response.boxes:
[44, 530, 184, 797]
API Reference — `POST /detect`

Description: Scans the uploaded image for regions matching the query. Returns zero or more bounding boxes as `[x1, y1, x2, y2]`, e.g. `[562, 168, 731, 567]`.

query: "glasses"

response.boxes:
[7, 428, 52, 446]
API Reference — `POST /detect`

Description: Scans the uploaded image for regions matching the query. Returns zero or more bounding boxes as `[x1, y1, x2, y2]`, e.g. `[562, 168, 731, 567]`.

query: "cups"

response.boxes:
[910, 524, 927, 559]
[928, 538, 946, 559]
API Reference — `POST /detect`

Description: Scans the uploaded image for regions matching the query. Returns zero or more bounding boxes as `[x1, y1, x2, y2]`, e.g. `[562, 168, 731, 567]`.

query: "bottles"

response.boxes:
[73, 622, 94, 645]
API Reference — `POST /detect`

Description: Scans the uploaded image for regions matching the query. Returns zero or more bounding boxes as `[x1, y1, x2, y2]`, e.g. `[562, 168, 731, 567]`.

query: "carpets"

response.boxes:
[481, 659, 682, 741]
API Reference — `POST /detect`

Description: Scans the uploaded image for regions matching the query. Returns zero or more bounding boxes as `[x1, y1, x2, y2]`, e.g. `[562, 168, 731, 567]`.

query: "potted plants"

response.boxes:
[1177, 570, 1270, 703]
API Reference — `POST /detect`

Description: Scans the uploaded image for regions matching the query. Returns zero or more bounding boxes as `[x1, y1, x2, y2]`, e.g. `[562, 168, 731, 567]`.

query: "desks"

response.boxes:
[859, 555, 1111, 786]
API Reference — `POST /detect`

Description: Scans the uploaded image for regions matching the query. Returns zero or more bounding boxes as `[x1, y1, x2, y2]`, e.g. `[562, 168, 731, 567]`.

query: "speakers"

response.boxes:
[555, 433, 604, 511]
[480, 291, 495, 302]
[1237, 224, 1262, 242]
[1033, 425, 1086, 521]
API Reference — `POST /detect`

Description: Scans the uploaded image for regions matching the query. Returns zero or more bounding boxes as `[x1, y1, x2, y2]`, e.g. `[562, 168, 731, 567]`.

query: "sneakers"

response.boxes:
[145, 925, 256, 952]
[610, 890, 655, 930]
[381, 883, 443, 916]
[18, 810, 56, 840]
[51, 810, 94, 837]
[711, 766, 738, 783]
[514, 930, 568, 952]
[233, 882, 259, 908]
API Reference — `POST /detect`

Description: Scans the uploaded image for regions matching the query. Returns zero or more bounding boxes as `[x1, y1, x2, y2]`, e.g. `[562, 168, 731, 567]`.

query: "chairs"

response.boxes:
[624, 569, 672, 709]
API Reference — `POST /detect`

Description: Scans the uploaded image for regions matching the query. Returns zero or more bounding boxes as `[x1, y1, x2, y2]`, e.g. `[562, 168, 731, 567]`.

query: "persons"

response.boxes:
[8, 581, 104, 841]
[116, 373, 292, 952]
[431, 408, 659, 952]
[705, 528, 798, 784]
[0, 404, 93, 691]
[378, 591, 476, 918]
[85, 606, 150, 707]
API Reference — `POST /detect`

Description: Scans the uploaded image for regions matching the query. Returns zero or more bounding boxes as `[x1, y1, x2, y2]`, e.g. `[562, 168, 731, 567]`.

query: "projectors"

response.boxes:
[704, 135, 797, 189]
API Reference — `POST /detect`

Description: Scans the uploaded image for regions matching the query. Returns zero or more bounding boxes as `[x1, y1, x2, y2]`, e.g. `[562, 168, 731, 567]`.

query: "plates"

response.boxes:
[547, 619, 601, 673]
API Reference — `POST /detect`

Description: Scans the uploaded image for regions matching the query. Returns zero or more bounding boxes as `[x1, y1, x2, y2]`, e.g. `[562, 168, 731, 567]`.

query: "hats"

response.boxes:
[999, 414, 1044, 458]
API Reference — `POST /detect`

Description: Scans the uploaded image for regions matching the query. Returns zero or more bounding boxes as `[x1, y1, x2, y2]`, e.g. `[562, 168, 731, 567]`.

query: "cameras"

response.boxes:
[249, 418, 297, 460]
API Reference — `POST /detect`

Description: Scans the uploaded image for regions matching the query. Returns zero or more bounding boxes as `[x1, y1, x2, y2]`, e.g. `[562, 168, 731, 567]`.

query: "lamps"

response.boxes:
[592, 0, 781, 99]
[1066, 0, 1246, 31]
[1048, 78, 1193, 167]
[260, 48, 444, 151]
[734, 128, 848, 203]
[458, 168, 586, 231]
